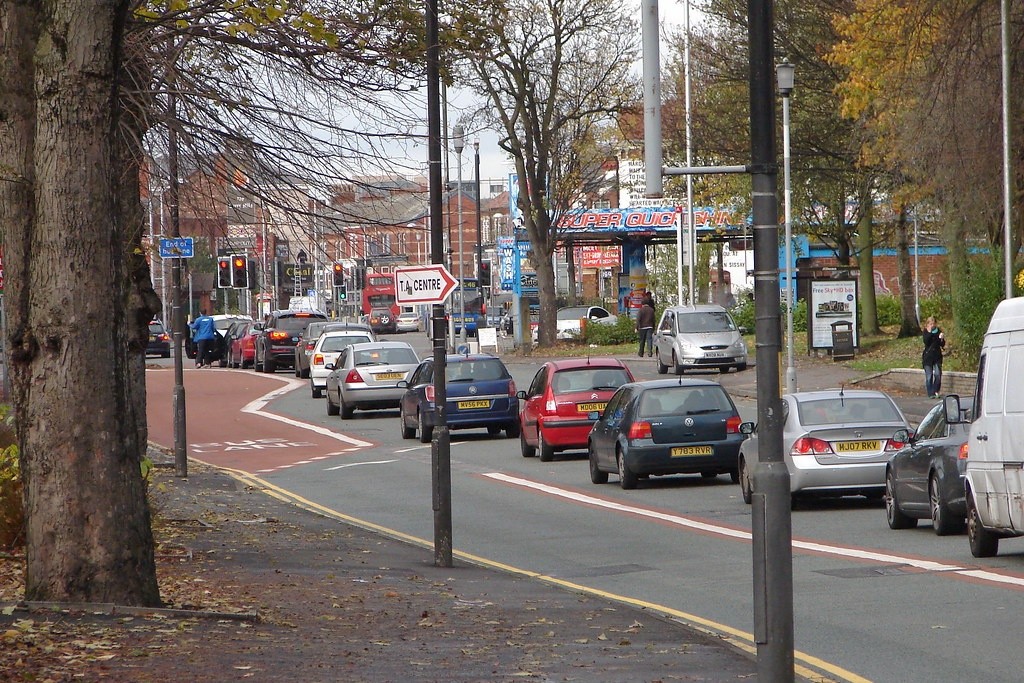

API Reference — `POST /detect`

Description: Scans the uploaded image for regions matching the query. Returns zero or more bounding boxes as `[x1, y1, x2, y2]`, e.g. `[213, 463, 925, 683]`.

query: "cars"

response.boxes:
[396, 354, 520, 442]
[883, 399, 973, 535]
[485, 304, 514, 333]
[584, 379, 746, 487]
[516, 356, 635, 462]
[533, 306, 618, 344]
[735, 393, 914, 507]
[396, 311, 420, 332]
[146, 309, 420, 420]
[655, 303, 747, 374]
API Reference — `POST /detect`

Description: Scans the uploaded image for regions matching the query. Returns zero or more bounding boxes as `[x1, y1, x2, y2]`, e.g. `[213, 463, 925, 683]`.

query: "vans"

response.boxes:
[941, 297, 1024, 556]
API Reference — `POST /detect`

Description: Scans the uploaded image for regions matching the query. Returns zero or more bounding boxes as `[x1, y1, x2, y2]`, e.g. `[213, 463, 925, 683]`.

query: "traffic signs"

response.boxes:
[394, 266, 458, 304]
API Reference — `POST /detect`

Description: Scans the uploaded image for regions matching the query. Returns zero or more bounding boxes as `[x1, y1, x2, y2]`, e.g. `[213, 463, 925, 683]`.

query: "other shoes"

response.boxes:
[934, 392, 940, 398]
[196, 363, 201, 368]
[201, 364, 208, 369]
[930, 396, 935, 398]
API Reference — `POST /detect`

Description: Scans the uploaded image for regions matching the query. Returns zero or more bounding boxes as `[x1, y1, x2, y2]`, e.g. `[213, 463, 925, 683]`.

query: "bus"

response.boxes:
[364, 273, 400, 316]
[444, 280, 485, 337]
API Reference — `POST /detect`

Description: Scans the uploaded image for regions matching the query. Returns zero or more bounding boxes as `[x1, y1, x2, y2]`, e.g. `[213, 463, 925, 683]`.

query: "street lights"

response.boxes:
[453, 124, 468, 343]
[775, 56, 798, 393]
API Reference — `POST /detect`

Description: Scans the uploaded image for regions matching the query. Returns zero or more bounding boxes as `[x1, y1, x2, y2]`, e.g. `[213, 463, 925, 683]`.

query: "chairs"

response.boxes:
[556, 376, 571, 391]
[601, 369, 615, 386]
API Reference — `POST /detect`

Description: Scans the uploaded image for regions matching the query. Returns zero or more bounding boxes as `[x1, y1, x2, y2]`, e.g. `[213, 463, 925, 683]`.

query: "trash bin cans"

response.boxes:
[830, 320, 854, 362]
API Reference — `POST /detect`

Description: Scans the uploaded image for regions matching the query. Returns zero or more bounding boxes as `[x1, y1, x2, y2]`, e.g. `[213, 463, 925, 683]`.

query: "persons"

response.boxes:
[187, 308, 215, 369]
[922, 317, 945, 399]
[635, 298, 655, 357]
[640, 292, 655, 312]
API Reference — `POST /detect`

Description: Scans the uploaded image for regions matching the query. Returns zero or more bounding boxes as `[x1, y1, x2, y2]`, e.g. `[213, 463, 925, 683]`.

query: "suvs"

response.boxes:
[368, 308, 394, 332]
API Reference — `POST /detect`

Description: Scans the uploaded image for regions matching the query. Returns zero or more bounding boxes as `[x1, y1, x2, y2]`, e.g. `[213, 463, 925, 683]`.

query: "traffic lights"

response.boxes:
[334, 263, 347, 303]
[481, 260, 492, 288]
[218, 255, 249, 289]
